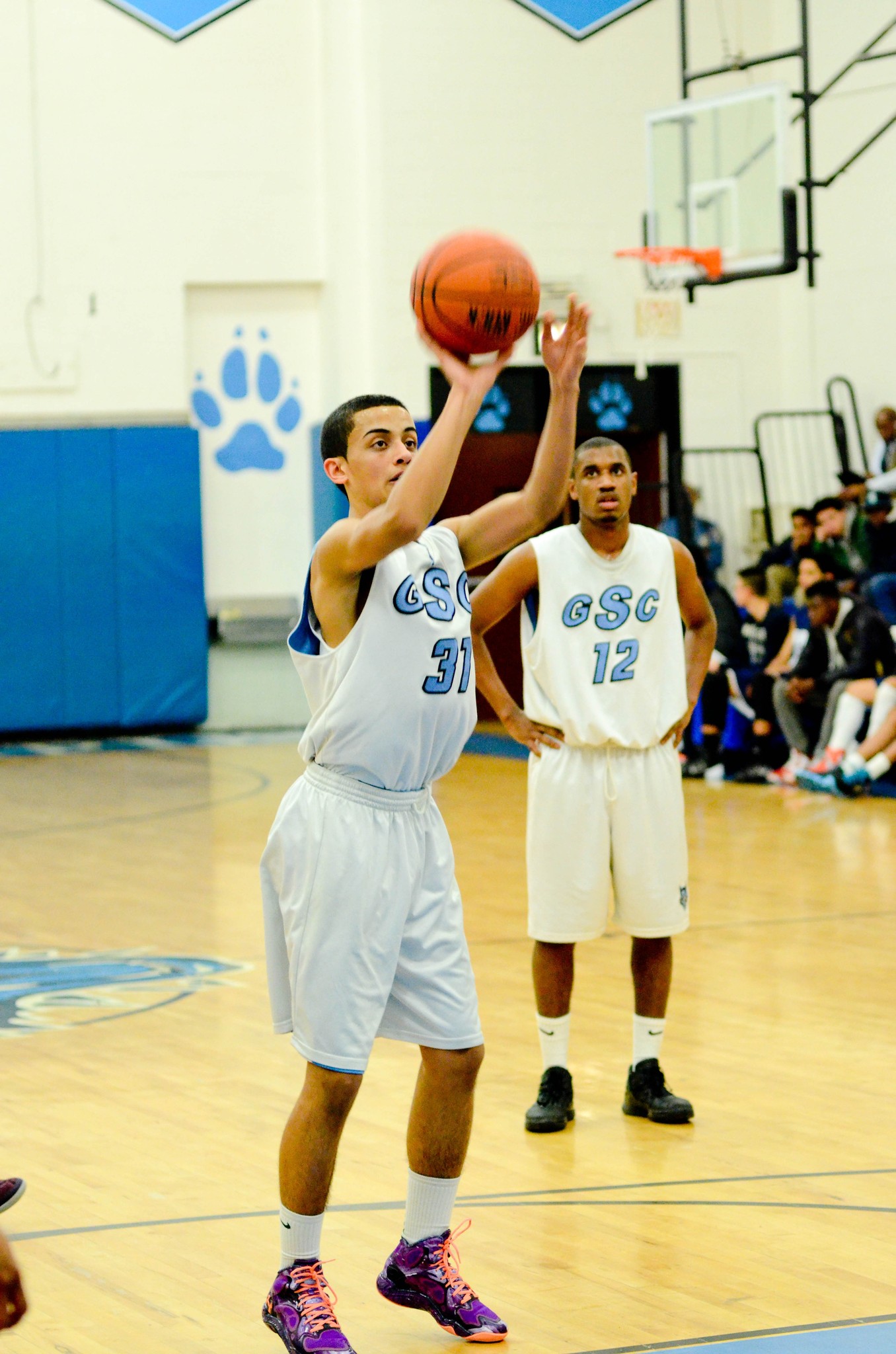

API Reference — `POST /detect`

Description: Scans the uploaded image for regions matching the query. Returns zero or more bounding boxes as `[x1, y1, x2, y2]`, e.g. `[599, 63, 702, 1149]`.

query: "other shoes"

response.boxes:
[0, 1178, 26, 1213]
[678, 746, 882, 799]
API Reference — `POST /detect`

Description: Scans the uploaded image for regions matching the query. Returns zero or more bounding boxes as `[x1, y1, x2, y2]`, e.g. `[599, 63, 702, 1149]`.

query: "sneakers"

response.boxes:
[524, 1066, 579, 1132]
[621, 1058, 695, 1124]
[375, 1218, 508, 1342]
[262, 1259, 358, 1354]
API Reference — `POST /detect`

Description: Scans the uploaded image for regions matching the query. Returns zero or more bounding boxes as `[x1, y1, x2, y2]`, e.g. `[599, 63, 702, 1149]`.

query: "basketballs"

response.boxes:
[410, 226, 541, 355]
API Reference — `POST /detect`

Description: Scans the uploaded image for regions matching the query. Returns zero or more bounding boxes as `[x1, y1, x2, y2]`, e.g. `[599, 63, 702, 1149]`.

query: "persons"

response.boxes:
[655, 407, 896, 801]
[261, 293, 588, 1354]
[469, 437, 718, 1125]
[0, 1178, 28, 1331]
